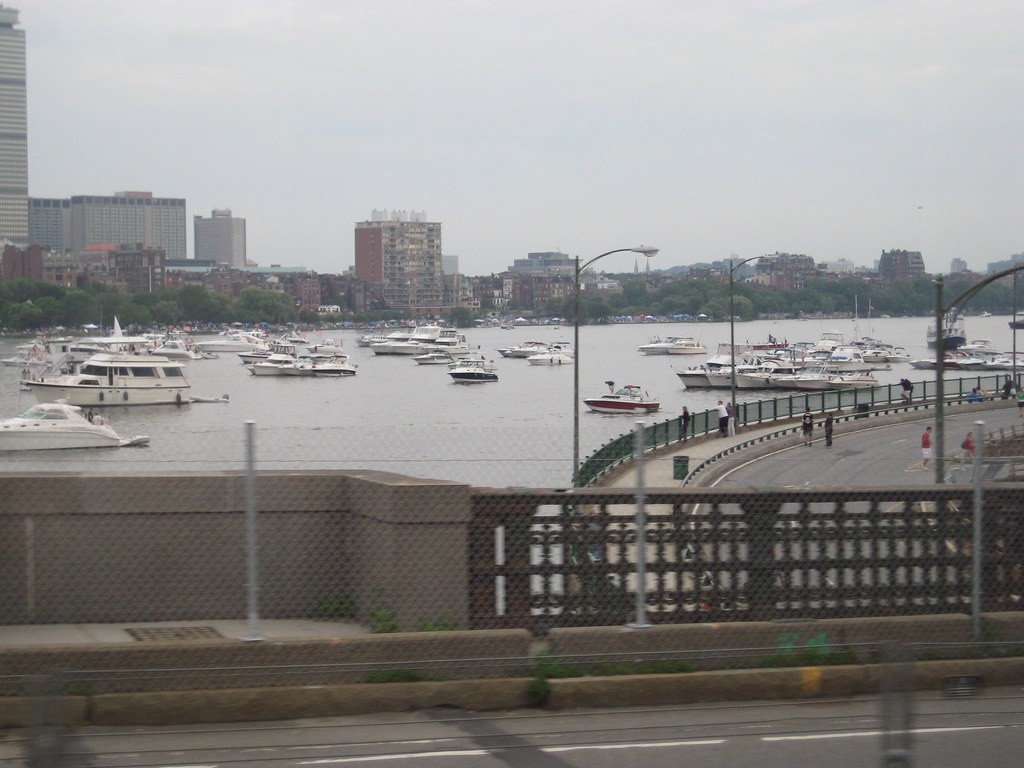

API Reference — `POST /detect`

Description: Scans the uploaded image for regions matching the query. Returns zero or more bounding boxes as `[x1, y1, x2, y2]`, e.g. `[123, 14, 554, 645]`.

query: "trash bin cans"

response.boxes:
[672, 454, 689, 480]
[856, 402, 869, 420]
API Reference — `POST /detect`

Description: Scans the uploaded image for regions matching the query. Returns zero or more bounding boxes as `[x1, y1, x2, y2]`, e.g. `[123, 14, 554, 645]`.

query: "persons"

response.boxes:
[726, 402, 735, 437]
[80, 408, 104, 426]
[825, 413, 834, 447]
[1000, 380, 1018, 400]
[800, 407, 815, 446]
[605, 381, 615, 395]
[898, 378, 914, 406]
[1015, 387, 1024, 417]
[713, 401, 729, 438]
[679, 406, 691, 443]
[959, 432, 976, 469]
[921, 426, 933, 470]
[965, 386, 989, 403]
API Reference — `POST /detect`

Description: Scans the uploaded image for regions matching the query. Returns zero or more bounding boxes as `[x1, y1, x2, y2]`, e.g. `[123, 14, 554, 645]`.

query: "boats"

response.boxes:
[925, 308, 967, 349]
[1017, 311, 1024, 316]
[909, 338, 1024, 372]
[528, 347, 573, 367]
[356, 324, 472, 355]
[679, 332, 912, 390]
[446, 360, 498, 384]
[943, 307, 965, 321]
[1008, 319, 1024, 331]
[582, 385, 661, 414]
[666, 339, 707, 355]
[446, 352, 498, 370]
[638, 336, 692, 355]
[980, 311, 991, 318]
[0, 314, 359, 451]
[497, 339, 575, 358]
[411, 349, 454, 365]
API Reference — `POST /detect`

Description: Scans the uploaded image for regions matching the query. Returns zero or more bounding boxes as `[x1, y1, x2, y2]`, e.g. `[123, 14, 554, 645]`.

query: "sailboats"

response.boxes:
[847, 291, 893, 352]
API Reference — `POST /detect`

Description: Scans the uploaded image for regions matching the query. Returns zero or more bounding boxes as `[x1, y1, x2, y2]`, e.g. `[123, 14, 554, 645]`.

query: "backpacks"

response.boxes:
[960, 441, 968, 449]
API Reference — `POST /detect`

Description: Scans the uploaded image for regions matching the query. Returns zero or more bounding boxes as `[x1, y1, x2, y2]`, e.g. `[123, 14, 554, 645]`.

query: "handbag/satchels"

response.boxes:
[800, 430, 804, 439]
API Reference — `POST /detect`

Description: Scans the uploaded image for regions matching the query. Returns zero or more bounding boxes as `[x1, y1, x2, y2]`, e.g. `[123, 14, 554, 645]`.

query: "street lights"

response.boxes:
[573, 244, 660, 468]
[729, 254, 779, 433]
